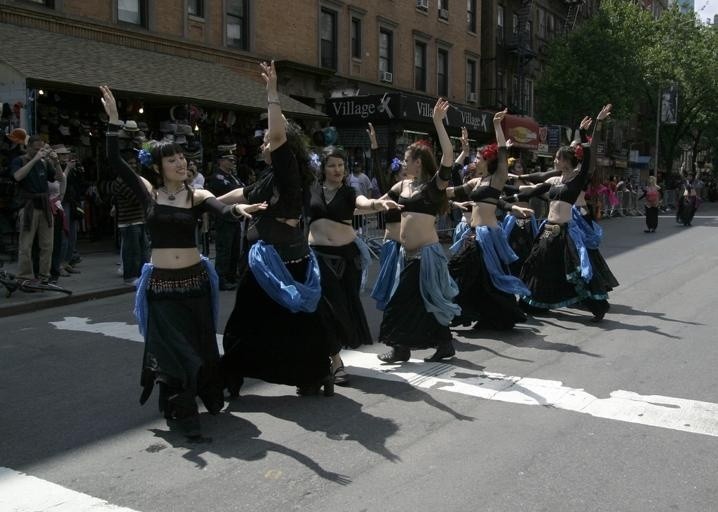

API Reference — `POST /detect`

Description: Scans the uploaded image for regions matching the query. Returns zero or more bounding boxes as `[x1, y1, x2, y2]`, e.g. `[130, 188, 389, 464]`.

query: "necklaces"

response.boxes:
[158, 183, 186, 200]
[322, 180, 344, 202]
[480, 175, 489, 185]
[407, 181, 426, 197]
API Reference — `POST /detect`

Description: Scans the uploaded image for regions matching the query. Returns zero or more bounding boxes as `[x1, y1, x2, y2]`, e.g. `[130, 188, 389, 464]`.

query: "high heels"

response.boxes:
[223, 380, 244, 399]
[297, 375, 334, 397]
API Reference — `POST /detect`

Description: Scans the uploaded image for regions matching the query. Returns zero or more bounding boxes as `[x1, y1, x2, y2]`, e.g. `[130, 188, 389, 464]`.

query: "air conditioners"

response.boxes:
[381, 71, 393, 83]
[416, 0, 429, 9]
[439, 8, 449, 20]
[469, 93, 477, 104]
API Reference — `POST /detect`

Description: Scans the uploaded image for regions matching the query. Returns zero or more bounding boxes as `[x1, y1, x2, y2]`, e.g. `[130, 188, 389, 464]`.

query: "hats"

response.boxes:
[217, 149, 236, 161]
[123, 121, 137, 132]
[139, 123, 148, 131]
[6, 128, 30, 145]
[353, 160, 362, 167]
[50, 144, 70, 155]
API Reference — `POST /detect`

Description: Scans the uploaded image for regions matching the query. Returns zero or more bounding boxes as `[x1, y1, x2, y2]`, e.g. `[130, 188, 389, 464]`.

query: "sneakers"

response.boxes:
[424, 344, 455, 363]
[183, 424, 203, 440]
[377, 346, 411, 362]
[122, 278, 140, 285]
[591, 302, 609, 323]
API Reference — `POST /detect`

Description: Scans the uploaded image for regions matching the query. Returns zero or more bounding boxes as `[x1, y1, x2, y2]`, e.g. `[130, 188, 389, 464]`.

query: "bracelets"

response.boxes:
[230, 203, 243, 218]
[370, 199, 376, 210]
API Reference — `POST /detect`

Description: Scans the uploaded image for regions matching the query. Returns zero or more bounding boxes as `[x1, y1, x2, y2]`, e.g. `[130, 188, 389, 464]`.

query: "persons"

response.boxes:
[100, 83, 267, 437]
[355, 97, 462, 363]
[207, 143, 246, 292]
[586, 169, 636, 217]
[215, 59, 336, 399]
[100, 152, 150, 288]
[185, 169, 203, 191]
[0, 126, 89, 291]
[637, 176, 663, 233]
[669, 170, 705, 226]
[300, 145, 405, 385]
[345, 161, 373, 230]
[266, 97, 280, 102]
[445, 103, 620, 332]
[366, 122, 470, 312]
[373, 159, 393, 230]
[236, 164, 257, 253]
[187, 160, 205, 189]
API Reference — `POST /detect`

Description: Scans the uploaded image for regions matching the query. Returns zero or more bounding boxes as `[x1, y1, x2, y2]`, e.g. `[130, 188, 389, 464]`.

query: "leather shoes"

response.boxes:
[219, 282, 236, 291]
[332, 364, 349, 384]
[59, 269, 69, 277]
[67, 266, 80, 274]
